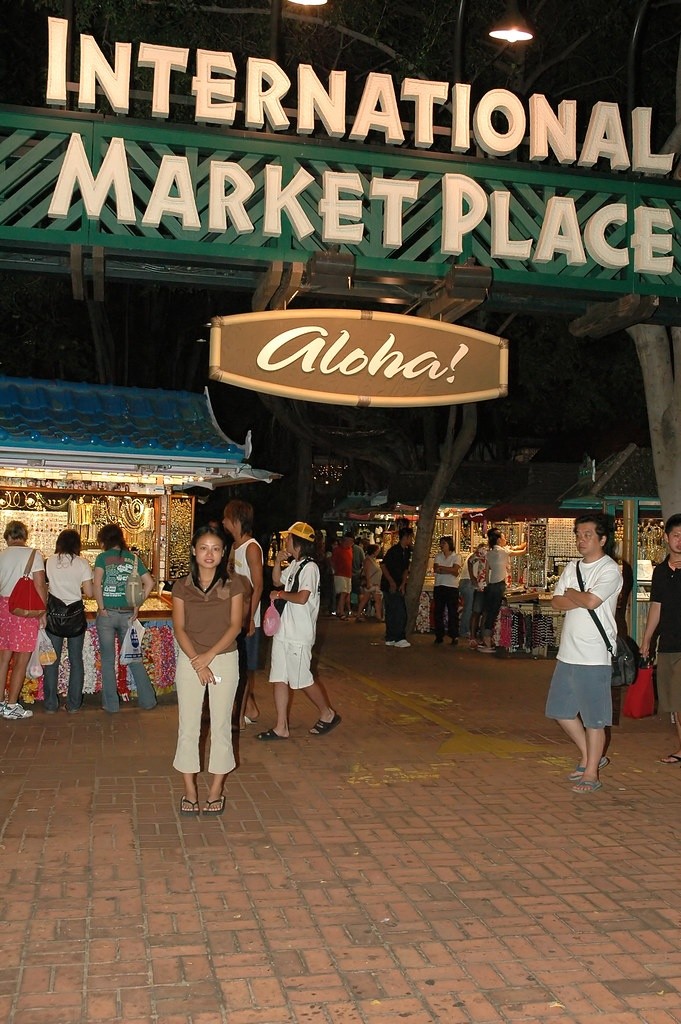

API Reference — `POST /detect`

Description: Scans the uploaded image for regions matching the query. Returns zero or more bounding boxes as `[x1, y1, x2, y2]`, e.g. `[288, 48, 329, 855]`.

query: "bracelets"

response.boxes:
[278, 591, 282, 599]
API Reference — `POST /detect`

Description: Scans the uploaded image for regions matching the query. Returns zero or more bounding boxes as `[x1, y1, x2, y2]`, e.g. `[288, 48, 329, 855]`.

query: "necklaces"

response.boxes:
[203, 589, 206, 592]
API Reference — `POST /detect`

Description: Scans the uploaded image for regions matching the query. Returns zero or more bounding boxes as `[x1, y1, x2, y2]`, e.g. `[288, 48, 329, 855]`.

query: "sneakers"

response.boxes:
[2, 703, 33, 719]
[0, 699, 7, 715]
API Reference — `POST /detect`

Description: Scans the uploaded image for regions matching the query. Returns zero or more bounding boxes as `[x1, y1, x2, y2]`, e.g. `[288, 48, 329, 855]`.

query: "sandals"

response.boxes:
[660, 754, 681, 763]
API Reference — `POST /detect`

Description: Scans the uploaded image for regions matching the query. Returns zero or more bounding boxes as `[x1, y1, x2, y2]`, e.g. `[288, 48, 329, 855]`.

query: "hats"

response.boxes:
[278, 522, 315, 542]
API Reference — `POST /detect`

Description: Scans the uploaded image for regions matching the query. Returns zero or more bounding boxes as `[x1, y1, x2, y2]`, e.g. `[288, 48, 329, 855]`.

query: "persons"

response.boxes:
[44, 530, 92, 713]
[545, 512, 624, 795]
[0, 521, 48, 719]
[433, 537, 461, 645]
[317, 529, 385, 621]
[256, 522, 341, 741]
[171, 528, 244, 816]
[639, 514, 681, 769]
[460, 528, 527, 652]
[222, 500, 264, 732]
[381, 528, 413, 646]
[93, 524, 158, 712]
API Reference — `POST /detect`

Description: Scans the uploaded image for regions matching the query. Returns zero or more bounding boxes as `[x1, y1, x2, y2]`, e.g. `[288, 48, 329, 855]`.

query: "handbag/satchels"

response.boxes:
[360, 575, 367, 587]
[623, 657, 655, 718]
[125, 554, 145, 607]
[40, 629, 57, 665]
[611, 632, 639, 686]
[25, 630, 44, 678]
[119, 617, 146, 665]
[8, 549, 46, 618]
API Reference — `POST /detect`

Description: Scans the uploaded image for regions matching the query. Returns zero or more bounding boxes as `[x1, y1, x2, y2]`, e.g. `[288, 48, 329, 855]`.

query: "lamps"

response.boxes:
[442, 259, 498, 303]
[453, 0, 532, 82]
[304, 245, 357, 291]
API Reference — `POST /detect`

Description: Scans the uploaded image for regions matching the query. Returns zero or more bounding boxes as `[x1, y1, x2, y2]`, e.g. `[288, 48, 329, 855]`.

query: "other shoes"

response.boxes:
[385, 639, 411, 647]
[60, 703, 78, 713]
[433, 637, 497, 653]
[331, 610, 386, 623]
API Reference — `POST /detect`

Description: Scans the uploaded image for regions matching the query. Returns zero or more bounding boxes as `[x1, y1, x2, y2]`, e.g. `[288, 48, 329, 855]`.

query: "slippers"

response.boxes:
[233, 715, 258, 731]
[568, 757, 610, 780]
[309, 714, 342, 736]
[571, 781, 602, 793]
[257, 729, 288, 739]
[180, 795, 200, 816]
[202, 796, 225, 815]
